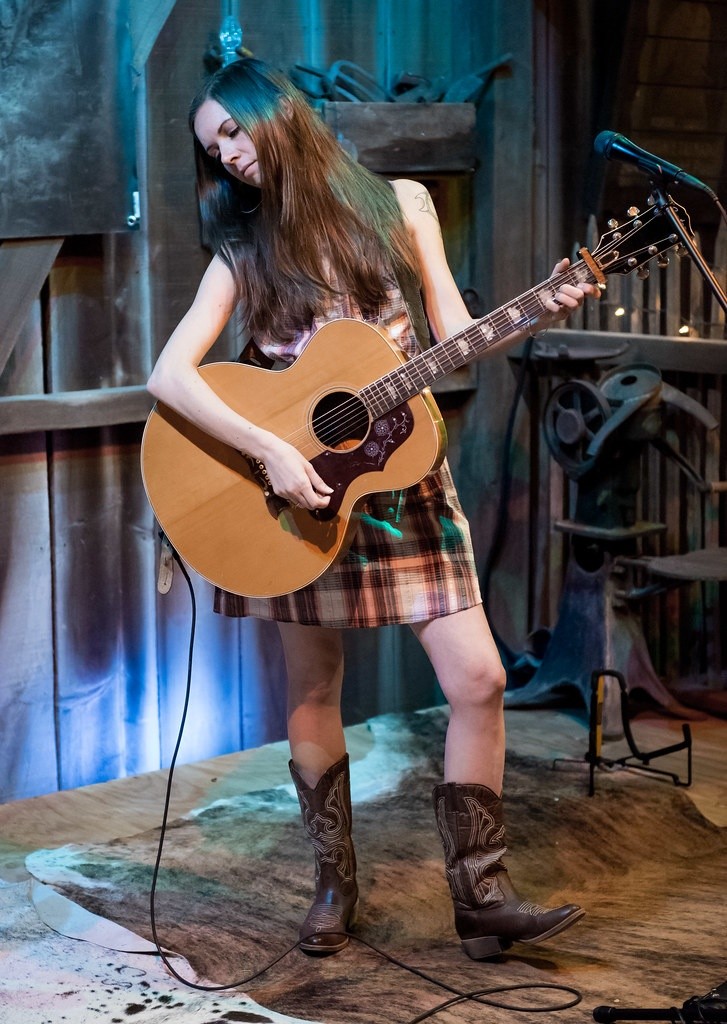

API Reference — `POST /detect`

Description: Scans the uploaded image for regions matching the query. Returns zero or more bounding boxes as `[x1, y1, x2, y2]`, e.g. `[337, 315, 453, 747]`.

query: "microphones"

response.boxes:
[594, 130, 707, 190]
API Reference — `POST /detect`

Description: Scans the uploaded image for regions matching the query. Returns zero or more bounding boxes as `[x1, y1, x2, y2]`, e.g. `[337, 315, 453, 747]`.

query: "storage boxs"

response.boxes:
[312, 100, 477, 173]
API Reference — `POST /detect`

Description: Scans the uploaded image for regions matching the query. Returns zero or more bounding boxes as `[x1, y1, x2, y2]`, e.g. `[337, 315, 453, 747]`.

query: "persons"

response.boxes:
[147, 58, 602, 961]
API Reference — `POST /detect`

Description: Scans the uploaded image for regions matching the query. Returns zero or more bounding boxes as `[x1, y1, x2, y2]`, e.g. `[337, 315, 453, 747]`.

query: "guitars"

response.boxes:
[138, 184, 703, 602]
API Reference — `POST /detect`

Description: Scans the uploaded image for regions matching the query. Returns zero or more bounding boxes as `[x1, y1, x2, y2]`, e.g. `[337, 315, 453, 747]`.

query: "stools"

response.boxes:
[589, 549, 727, 769]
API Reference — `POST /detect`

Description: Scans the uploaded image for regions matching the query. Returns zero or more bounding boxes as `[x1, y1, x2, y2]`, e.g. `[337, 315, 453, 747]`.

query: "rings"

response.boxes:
[296, 503, 300, 507]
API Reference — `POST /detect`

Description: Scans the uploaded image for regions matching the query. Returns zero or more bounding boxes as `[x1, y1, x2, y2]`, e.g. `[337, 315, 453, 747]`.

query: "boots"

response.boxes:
[430, 782, 587, 961]
[288, 755, 361, 953]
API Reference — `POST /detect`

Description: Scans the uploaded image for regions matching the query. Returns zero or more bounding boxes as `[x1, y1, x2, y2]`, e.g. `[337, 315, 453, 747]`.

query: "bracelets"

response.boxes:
[527, 322, 549, 339]
